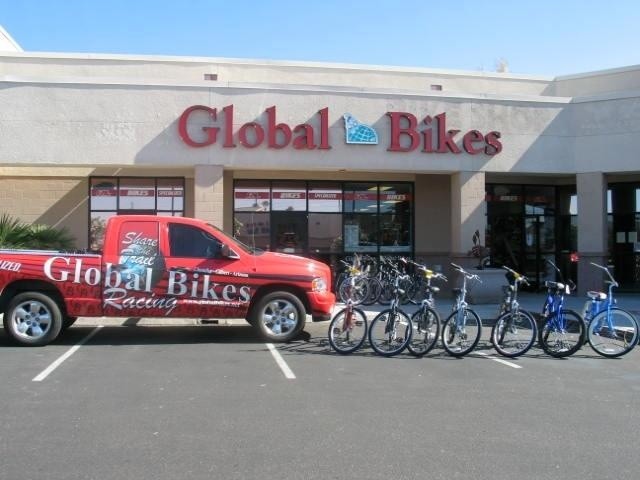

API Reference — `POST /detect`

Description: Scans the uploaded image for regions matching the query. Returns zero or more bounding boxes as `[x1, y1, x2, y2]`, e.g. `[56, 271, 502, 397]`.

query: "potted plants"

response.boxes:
[461, 229, 509, 305]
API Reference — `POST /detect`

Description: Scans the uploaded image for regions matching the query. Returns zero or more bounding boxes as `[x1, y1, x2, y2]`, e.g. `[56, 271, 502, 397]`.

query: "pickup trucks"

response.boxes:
[0, 215, 336, 346]
